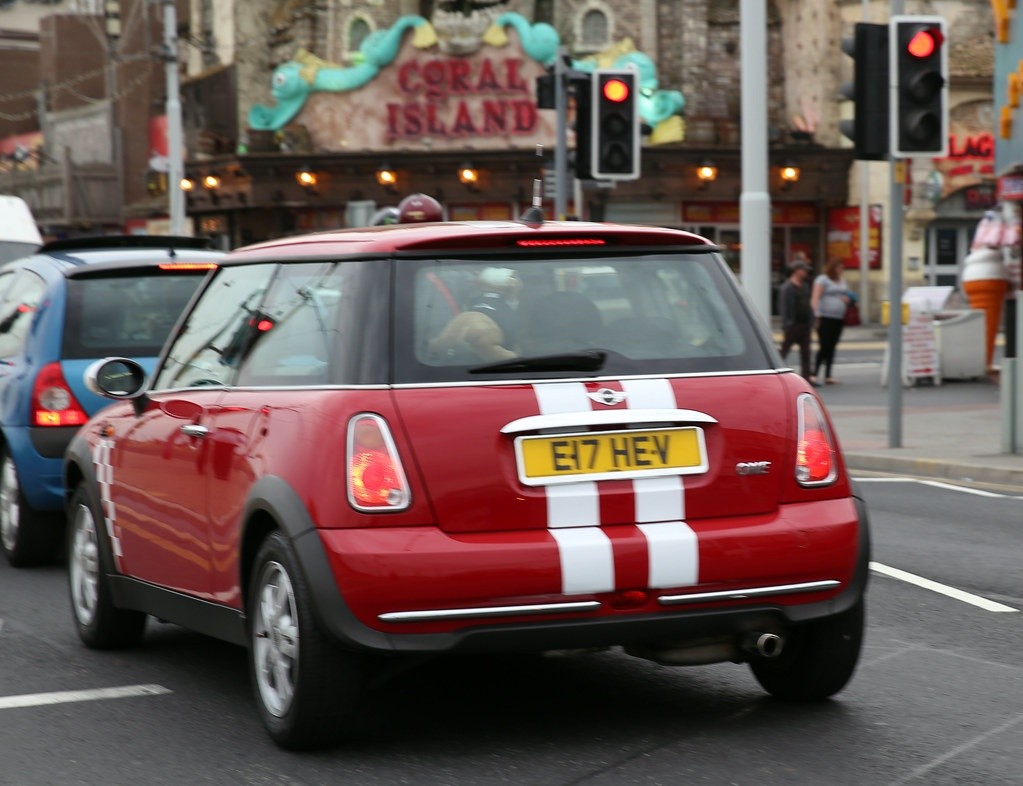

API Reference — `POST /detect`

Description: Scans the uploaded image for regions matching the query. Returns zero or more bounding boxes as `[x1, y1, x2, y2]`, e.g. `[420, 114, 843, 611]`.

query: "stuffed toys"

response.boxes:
[428, 267, 524, 364]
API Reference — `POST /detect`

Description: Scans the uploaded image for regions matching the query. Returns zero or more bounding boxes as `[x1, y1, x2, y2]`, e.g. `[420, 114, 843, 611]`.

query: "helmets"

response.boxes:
[369, 207, 399, 225]
[399, 194, 443, 222]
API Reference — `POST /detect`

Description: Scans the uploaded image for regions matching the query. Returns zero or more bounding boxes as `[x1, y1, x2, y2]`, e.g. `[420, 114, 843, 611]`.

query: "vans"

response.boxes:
[0, 195, 44, 265]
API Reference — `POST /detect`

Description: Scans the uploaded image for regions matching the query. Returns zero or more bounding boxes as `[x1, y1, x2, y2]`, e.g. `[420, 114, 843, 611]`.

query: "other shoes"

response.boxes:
[823, 378, 841, 385]
[809, 380, 822, 387]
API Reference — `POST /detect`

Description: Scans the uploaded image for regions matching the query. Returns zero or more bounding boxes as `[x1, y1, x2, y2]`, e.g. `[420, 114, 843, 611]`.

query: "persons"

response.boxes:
[808, 258, 853, 384]
[779, 251, 814, 387]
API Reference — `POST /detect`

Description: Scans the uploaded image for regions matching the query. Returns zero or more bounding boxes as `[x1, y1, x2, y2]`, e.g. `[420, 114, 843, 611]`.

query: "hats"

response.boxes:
[790, 260, 813, 271]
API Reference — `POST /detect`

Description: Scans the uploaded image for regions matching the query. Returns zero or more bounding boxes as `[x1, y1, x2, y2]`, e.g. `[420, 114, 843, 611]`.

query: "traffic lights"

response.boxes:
[590, 70, 640, 180]
[889, 13, 951, 159]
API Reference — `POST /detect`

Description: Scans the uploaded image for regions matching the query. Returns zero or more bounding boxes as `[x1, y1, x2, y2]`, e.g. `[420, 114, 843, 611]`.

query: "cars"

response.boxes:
[0, 231, 228, 569]
[61, 218, 870, 752]
